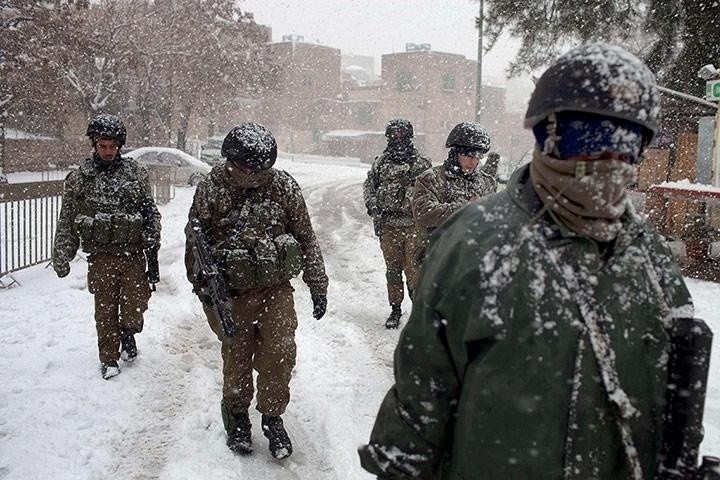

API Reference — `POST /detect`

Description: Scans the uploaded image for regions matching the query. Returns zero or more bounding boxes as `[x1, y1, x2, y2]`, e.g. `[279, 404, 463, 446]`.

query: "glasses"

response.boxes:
[389, 132, 407, 139]
[458, 149, 485, 160]
[531, 114, 644, 163]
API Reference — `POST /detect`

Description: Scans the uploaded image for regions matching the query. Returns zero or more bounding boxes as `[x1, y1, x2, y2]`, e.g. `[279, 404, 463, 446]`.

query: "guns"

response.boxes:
[142, 195, 161, 283]
[186, 209, 238, 336]
[656, 317, 719, 480]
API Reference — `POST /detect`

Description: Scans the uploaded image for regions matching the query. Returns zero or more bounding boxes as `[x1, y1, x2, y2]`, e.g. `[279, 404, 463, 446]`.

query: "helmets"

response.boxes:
[220, 122, 277, 171]
[446, 121, 490, 154]
[86, 114, 127, 144]
[385, 118, 414, 137]
[524, 42, 661, 141]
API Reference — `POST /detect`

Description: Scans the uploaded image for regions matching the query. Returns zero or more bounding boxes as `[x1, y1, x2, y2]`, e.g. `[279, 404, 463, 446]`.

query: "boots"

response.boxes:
[226, 417, 253, 455]
[261, 414, 293, 459]
[102, 360, 121, 379]
[384, 304, 402, 328]
[119, 327, 137, 361]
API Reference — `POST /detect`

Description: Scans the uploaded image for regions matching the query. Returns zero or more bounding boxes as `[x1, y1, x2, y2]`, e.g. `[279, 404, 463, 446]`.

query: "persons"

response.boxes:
[412, 120, 500, 238]
[357, 44, 708, 479]
[184, 118, 328, 463]
[50, 114, 162, 382]
[362, 108, 434, 330]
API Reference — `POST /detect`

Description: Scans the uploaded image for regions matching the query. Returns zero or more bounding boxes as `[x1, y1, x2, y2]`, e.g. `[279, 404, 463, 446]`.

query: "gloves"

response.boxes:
[311, 293, 327, 318]
[145, 238, 161, 253]
[52, 248, 71, 278]
[365, 199, 380, 216]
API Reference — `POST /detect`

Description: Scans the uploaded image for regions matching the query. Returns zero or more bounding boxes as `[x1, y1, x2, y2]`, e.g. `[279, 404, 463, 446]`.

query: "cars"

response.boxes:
[121, 146, 212, 188]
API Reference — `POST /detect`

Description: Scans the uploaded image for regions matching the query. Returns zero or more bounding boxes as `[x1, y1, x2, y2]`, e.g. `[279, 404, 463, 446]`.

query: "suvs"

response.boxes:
[200, 137, 226, 164]
